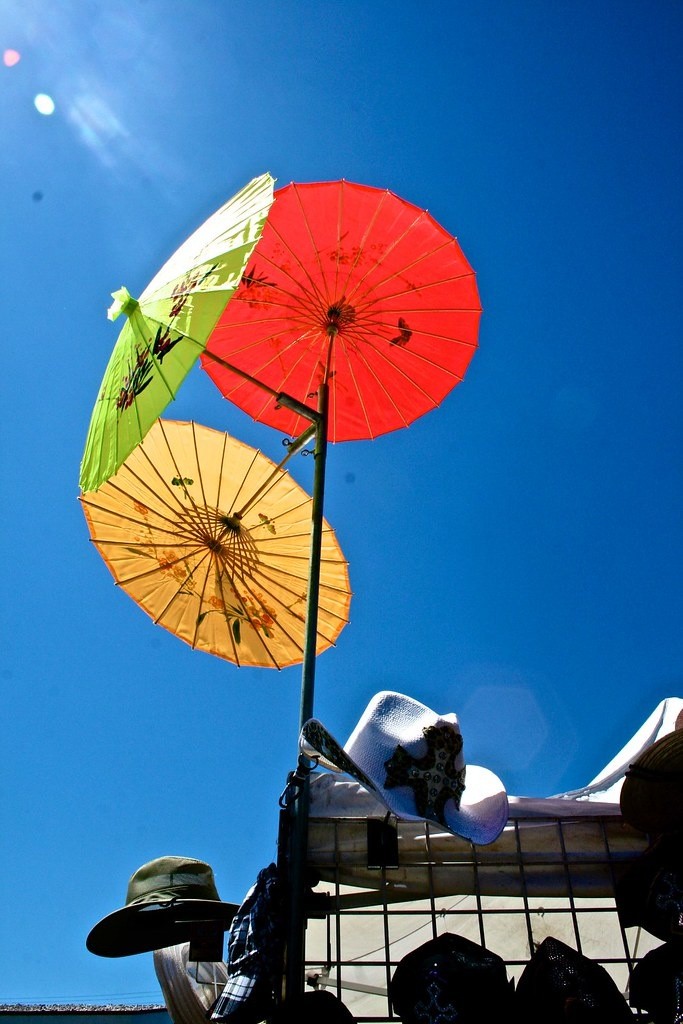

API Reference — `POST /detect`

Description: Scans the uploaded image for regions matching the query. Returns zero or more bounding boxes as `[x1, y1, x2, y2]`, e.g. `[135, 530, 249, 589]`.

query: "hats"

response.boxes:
[85, 855, 284, 1024]
[284, 990, 358, 1024]
[390, 933, 683, 1023]
[617, 846, 683, 942]
[298, 690, 511, 846]
[619, 710, 682, 839]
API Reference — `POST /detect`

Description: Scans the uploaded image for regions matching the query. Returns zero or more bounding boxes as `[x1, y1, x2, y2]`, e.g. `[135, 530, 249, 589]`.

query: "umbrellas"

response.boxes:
[196, 175, 485, 449]
[74, 168, 285, 493]
[74, 410, 356, 675]
